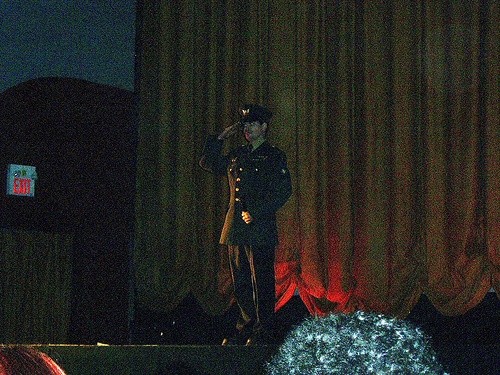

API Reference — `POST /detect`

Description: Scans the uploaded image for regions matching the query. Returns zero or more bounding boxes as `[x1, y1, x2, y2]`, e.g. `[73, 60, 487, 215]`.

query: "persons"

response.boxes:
[198, 104, 292, 346]
[260, 309, 449, 375]
[0, 344, 66, 375]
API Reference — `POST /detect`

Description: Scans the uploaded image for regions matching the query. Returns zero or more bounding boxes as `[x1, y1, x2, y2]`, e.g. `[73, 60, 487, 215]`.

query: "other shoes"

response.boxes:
[222, 332, 244, 346]
[244, 334, 272, 347]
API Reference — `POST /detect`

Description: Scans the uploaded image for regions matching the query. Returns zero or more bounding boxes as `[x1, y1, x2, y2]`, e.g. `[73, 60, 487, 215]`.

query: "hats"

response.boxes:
[240, 103, 273, 126]
